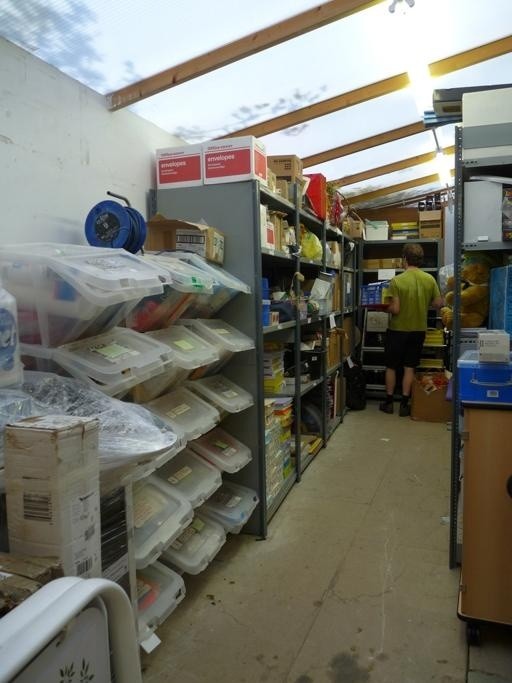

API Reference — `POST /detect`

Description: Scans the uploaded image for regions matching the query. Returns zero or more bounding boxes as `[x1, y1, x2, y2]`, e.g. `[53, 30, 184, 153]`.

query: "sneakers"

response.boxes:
[398, 405, 412, 417]
[378, 402, 393, 414]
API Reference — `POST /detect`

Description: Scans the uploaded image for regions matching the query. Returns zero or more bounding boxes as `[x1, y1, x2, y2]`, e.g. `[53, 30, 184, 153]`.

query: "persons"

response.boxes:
[376, 244, 442, 416]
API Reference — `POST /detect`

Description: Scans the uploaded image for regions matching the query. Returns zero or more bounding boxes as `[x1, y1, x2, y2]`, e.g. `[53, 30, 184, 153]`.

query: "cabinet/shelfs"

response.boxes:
[445, 119, 511, 566]
[154, 186, 361, 533]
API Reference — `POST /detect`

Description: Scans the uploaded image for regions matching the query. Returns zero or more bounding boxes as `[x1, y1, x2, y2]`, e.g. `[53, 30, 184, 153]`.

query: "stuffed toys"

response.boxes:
[439, 253, 492, 328]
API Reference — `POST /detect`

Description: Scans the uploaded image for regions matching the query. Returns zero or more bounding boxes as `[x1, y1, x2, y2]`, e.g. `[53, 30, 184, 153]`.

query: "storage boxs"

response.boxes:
[1, 409, 105, 617]
[0, 244, 259, 645]
[410, 372, 455, 423]
[155, 136, 356, 236]
[360, 206, 443, 350]
[304, 268, 356, 316]
[284, 317, 354, 380]
[456, 348, 512, 408]
[145, 213, 227, 263]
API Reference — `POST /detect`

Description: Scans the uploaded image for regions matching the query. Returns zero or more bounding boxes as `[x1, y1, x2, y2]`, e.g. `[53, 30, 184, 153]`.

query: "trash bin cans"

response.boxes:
[343, 369, 367, 411]
